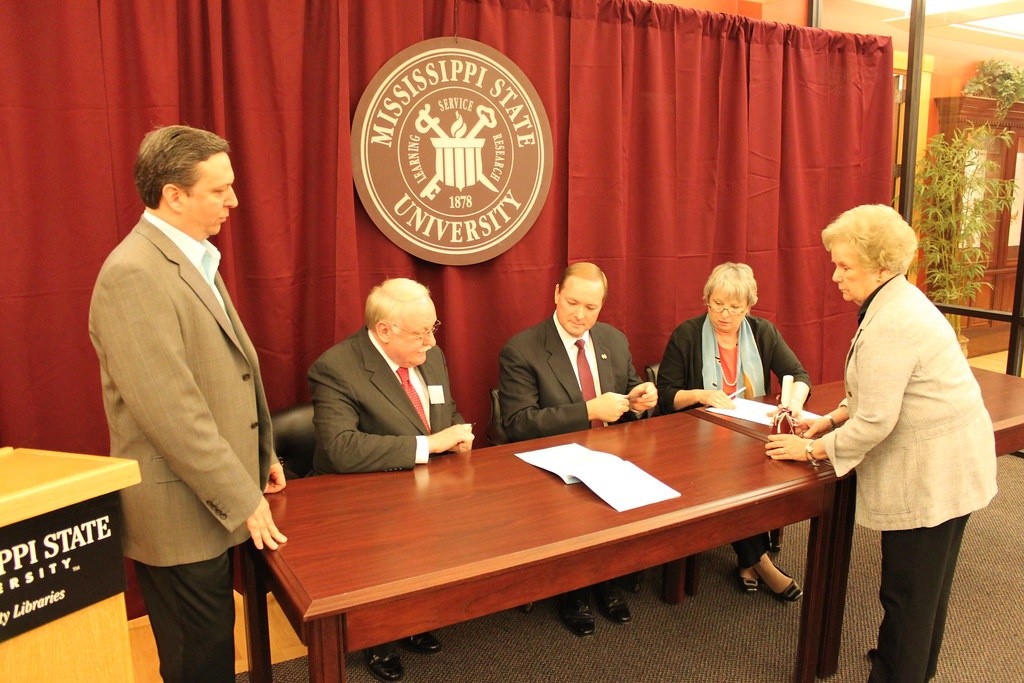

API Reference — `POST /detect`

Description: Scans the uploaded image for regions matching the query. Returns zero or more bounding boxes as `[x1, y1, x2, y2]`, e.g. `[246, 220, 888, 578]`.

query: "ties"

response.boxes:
[396, 366, 431, 435]
[575, 339, 604, 429]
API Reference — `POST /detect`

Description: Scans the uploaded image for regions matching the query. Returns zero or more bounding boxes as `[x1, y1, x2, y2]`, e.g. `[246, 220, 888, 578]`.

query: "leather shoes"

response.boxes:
[554, 587, 595, 637]
[590, 580, 631, 625]
[516, 602, 533, 615]
[617, 569, 646, 594]
[395, 631, 442, 655]
[365, 643, 404, 683]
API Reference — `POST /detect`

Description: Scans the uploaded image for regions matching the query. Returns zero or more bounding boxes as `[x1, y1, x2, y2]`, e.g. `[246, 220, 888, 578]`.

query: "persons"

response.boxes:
[306, 275, 476, 683]
[765, 205, 1000, 683]
[499, 262, 657, 634]
[657, 262, 812, 605]
[87, 125, 289, 683]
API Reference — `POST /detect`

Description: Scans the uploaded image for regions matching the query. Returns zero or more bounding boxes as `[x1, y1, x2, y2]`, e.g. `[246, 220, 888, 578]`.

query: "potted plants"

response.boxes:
[913, 122, 1020, 363]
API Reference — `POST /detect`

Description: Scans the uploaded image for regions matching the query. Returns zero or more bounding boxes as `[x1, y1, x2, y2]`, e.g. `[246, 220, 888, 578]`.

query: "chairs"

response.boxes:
[647, 363, 665, 416]
[486, 386, 636, 612]
[268, 400, 316, 480]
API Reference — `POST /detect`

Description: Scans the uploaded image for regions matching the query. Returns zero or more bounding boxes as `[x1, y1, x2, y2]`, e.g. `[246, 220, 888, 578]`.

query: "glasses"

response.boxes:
[375, 319, 441, 344]
[707, 294, 748, 315]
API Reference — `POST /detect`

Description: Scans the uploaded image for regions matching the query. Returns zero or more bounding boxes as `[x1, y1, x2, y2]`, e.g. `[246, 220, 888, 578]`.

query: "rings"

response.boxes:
[781, 448, 784, 453]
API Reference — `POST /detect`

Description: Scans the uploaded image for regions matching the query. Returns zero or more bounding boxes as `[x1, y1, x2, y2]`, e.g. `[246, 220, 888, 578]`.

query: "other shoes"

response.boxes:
[868, 648, 929, 683]
[754, 561, 804, 602]
[738, 565, 760, 594]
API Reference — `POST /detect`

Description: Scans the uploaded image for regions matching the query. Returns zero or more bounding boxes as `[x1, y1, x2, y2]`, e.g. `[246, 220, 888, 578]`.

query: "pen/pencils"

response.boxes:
[728, 387, 747, 398]
[625, 391, 646, 400]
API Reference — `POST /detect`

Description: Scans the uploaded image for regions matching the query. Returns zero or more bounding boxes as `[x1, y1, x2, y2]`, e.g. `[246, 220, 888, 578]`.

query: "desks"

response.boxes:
[240, 367, 1024, 683]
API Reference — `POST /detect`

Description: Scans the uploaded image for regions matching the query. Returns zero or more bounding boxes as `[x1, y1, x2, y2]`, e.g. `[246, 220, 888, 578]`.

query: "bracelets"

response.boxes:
[806, 440, 820, 466]
[827, 415, 836, 427]
